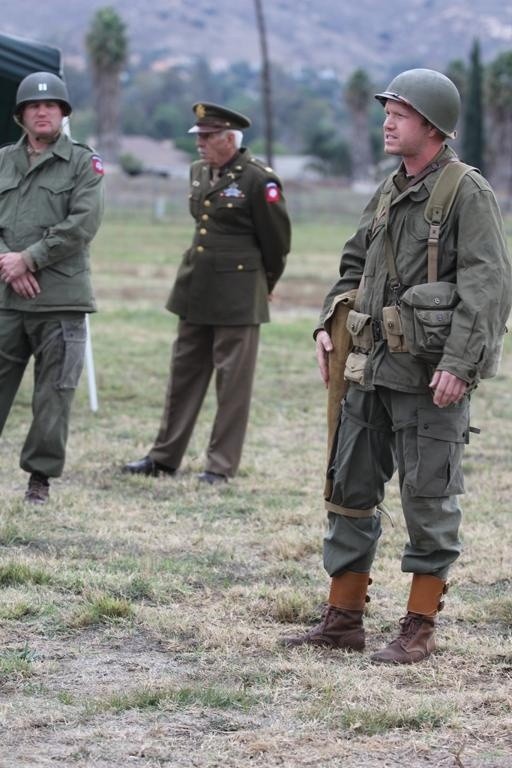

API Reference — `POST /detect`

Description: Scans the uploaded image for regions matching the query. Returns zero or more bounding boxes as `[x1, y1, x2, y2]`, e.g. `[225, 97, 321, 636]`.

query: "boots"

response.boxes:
[371, 612, 439, 666]
[19, 473, 55, 506]
[282, 605, 366, 650]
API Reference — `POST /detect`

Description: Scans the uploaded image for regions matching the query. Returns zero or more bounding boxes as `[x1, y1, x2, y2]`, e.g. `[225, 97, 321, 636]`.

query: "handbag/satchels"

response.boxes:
[401, 280, 462, 361]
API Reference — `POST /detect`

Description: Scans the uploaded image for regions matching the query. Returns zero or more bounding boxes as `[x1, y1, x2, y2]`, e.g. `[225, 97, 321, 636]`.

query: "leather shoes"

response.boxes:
[197, 466, 226, 484]
[122, 458, 176, 476]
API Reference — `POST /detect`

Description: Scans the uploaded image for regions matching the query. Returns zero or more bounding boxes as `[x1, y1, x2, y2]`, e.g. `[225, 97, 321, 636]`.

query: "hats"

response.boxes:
[187, 101, 252, 137]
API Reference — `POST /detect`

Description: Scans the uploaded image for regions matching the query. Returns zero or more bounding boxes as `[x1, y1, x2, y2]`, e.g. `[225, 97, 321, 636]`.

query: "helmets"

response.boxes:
[376, 67, 461, 141]
[14, 72, 72, 113]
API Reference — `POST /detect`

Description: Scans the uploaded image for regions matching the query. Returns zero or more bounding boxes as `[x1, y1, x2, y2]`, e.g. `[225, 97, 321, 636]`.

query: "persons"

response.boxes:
[277, 65, 512, 666]
[1, 70, 106, 502]
[117, 101, 293, 487]
